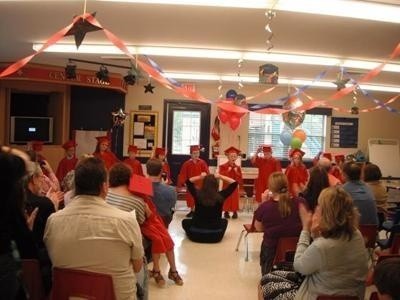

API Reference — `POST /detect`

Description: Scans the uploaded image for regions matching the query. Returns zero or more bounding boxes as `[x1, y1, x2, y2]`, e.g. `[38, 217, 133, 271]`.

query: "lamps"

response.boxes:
[63, 58, 77, 79]
[122, 69, 136, 86]
[95, 63, 112, 84]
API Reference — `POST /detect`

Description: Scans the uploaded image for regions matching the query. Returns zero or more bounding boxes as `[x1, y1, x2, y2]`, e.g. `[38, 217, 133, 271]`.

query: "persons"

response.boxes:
[56, 139, 81, 184]
[317, 158, 341, 188]
[340, 160, 381, 230]
[146, 158, 178, 229]
[249, 143, 284, 207]
[93, 136, 120, 172]
[256, 184, 369, 300]
[371, 256, 399, 300]
[21, 161, 57, 280]
[105, 162, 151, 224]
[63, 151, 95, 208]
[138, 195, 185, 288]
[312, 152, 333, 173]
[0, 145, 40, 259]
[27, 149, 61, 194]
[345, 154, 357, 163]
[218, 145, 243, 220]
[362, 162, 388, 231]
[284, 148, 309, 202]
[253, 170, 311, 278]
[185, 170, 238, 230]
[328, 154, 345, 184]
[42, 157, 149, 300]
[148, 147, 172, 186]
[32, 140, 46, 160]
[176, 145, 210, 218]
[122, 145, 145, 177]
[298, 164, 331, 214]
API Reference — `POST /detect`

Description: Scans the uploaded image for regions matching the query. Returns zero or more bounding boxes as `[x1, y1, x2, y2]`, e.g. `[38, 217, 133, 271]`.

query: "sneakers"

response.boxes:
[232, 214, 238, 219]
[224, 212, 230, 219]
[186, 211, 194, 217]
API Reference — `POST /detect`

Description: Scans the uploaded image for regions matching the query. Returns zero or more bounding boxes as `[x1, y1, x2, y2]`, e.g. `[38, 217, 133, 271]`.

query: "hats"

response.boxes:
[323, 152, 332, 161]
[32, 142, 45, 151]
[155, 148, 164, 155]
[128, 145, 137, 154]
[190, 145, 200, 153]
[335, 155, 345, 162]
[62, 141, 77, 150]
[129, 175, 154, 199]
[258, 145, 275, 153]
[95, 136, 111, 142]
[289, 148, 306, 157]
[225, 147, 241, 155]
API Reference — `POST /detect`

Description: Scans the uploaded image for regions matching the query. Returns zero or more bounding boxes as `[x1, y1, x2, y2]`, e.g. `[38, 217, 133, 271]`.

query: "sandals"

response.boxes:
[168, 269, 184, 286]
[151, 268, 165, 286]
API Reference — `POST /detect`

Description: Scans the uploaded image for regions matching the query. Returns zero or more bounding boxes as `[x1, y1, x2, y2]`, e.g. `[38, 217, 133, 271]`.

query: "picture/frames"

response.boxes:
[128, 109, 159, 158]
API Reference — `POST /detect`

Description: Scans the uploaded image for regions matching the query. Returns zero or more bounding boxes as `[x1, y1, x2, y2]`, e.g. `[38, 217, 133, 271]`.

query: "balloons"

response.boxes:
[281, 94, 307, 129]
[294, 128, 307, 143]
[217, 88, 249, 133]
[279, 129, 293, 148]
[290, 137, 303, 151]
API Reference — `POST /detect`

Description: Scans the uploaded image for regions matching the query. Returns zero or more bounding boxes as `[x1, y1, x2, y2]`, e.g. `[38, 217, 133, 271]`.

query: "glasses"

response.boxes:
[36, 175, 45, 181]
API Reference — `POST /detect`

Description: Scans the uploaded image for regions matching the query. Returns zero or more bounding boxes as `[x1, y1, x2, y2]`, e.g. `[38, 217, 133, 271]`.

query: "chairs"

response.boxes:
[50, 266, 118, 300]
[18, 257, 45, 300]
[235, 220, 269, 262]
[358, 220, 379, 256]
[272, 237, 300, 265]
